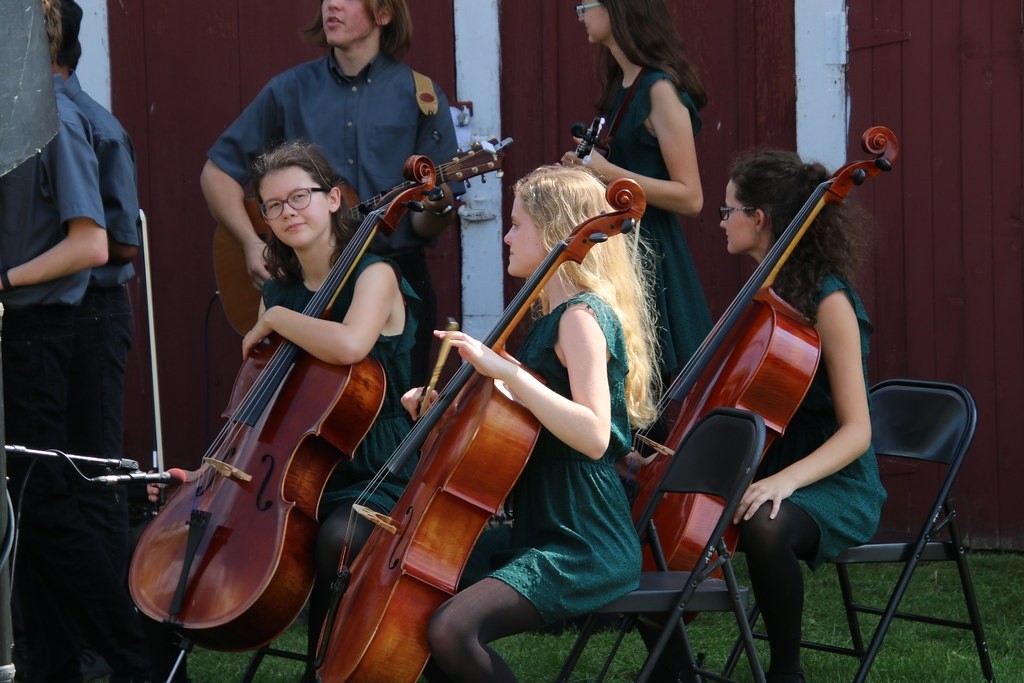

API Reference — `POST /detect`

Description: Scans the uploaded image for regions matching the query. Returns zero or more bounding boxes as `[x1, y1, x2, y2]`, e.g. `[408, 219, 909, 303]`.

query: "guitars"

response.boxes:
[206, 127, 522, 349]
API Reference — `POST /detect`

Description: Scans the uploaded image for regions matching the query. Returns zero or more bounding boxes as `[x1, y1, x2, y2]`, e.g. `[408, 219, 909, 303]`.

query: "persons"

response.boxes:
[199, 2, 461, 384]
[128, 137, 418, 683]
[399, 163, 644, 683]
[630, 150, 889, 680]
[0, 0, 189, 683]
[561, 0, 714, 399]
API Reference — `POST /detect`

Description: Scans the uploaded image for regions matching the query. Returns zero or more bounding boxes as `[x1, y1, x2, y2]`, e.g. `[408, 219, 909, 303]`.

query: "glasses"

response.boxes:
[259, 186, 326, 218]
[719, 204, 756, 220]
[575, 1, 600, 17]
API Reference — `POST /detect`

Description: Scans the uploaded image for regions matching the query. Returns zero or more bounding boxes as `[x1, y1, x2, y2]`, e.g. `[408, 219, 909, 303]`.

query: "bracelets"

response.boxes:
[422, 198, 454, 216]
[0, 266, 16, 290]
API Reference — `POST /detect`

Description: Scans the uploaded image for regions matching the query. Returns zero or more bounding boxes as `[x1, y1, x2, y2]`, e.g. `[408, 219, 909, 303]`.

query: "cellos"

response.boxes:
[598, 116, 908, 590]
[301, 167, 653, 683]
[122, 148, 446, 683]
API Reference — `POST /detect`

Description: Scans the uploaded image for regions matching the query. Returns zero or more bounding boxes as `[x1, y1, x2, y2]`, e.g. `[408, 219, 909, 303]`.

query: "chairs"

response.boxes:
[554, 407, 768, 683]
[721, 378, 995, 683]
[240, 602, 321, 683]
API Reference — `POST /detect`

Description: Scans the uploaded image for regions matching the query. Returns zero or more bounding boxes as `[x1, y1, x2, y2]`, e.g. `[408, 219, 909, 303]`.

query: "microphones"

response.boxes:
[91, 468, 186, 486]
